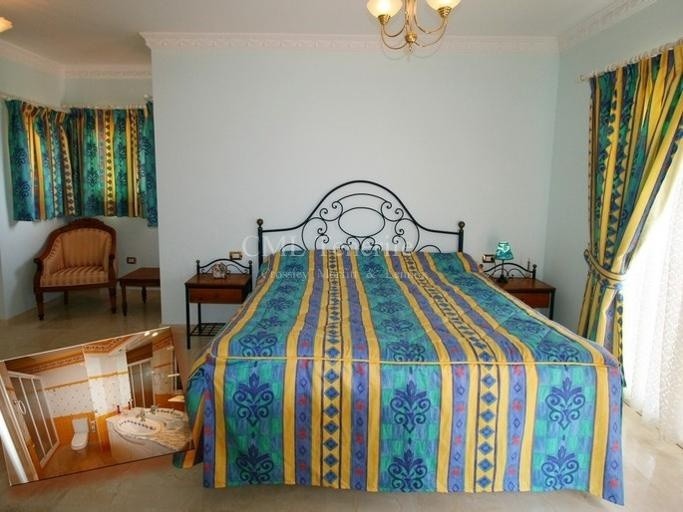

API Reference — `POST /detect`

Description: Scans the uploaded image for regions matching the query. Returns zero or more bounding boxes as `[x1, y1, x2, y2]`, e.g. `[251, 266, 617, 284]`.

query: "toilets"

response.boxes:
[69, 416, 89, 449]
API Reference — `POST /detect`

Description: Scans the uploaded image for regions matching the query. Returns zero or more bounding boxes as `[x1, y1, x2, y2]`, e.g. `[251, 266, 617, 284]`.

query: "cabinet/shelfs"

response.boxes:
[111, 432, 149, 461]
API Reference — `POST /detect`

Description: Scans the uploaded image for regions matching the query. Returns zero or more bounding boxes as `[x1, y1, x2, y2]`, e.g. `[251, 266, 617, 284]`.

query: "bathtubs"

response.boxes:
[116, 416, 160, 437]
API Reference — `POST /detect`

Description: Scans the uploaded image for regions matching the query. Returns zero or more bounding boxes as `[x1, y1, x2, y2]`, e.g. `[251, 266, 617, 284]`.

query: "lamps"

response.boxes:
[493, 241, 514, 283]
[366, 1, 464, 53]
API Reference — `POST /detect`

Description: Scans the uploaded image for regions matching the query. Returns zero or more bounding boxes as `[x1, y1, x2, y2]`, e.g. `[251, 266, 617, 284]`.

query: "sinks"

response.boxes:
[168, 395, 185, 402]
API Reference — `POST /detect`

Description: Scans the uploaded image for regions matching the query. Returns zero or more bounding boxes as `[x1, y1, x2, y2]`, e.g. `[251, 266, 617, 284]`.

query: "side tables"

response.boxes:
[118, 268, 159, 316]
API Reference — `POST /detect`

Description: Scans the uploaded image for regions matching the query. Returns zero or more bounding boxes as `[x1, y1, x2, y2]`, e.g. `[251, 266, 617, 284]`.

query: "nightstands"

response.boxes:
[478, 263, 556, 321]
[183, 257, 253, 350]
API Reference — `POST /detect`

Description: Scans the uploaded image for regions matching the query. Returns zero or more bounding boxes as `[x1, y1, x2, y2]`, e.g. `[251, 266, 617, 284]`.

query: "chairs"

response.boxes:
[31, 216, 120, 321]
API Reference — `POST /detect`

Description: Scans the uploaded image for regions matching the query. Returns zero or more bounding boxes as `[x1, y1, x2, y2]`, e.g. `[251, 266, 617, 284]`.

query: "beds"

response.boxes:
[194, 178, 625, 491]
